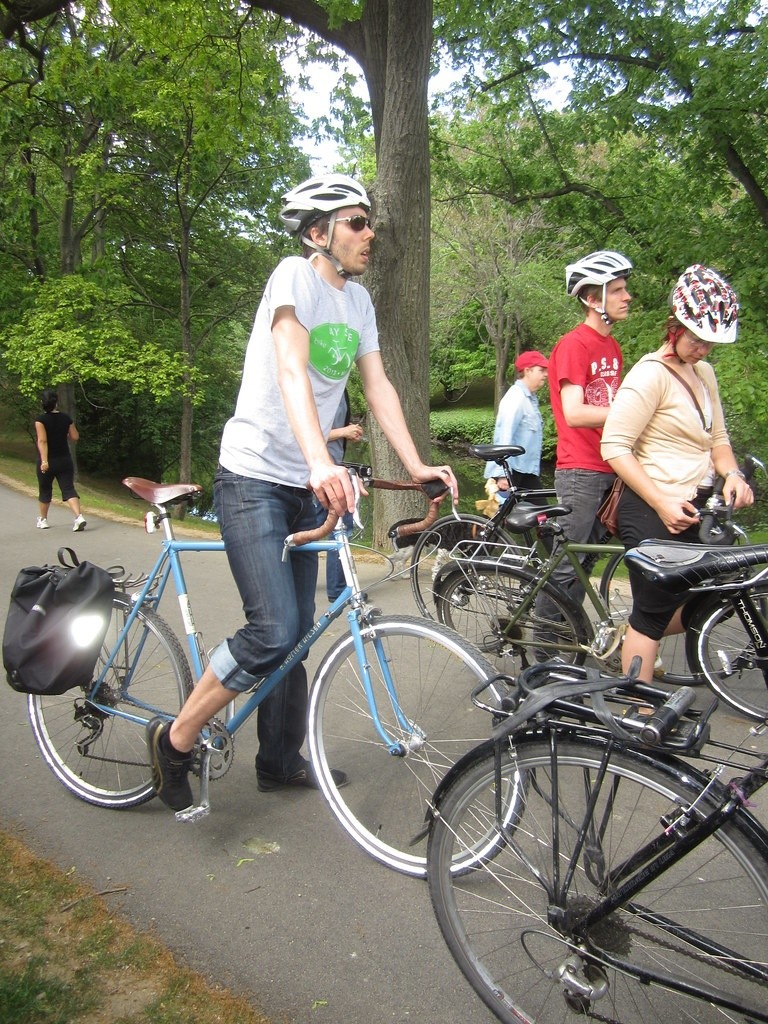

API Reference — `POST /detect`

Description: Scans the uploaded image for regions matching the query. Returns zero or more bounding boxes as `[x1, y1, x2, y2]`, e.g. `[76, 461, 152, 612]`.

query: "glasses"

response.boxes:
[326, 214, 371, 233]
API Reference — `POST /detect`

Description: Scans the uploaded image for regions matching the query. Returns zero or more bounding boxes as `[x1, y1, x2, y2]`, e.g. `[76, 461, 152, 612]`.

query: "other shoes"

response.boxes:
[329, 592, 369, 602]
[620, 625, 663, 669]
[558, 635, 594, 655]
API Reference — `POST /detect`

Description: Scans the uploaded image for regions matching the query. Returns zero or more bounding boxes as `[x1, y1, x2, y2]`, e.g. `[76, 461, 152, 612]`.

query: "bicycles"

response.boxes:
[404, 537, 768, 1024]
[23, 460, 535, 883]
[385, 444, 768, 729]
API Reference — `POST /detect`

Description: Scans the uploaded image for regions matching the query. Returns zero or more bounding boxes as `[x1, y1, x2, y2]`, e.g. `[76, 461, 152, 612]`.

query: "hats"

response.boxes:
[515, 351, 549, 372]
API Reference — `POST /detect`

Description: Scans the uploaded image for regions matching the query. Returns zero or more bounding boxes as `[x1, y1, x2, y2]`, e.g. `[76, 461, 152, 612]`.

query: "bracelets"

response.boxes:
[723, 467, 745, 480]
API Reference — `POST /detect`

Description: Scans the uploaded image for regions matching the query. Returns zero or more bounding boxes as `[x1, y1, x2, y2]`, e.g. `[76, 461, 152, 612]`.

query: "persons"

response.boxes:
[140, 172, 458, 812]
[312, 385, 368, 603]
[35, 391, 86, 531]
[533, 250, 634, 664]
[600, 265, 754, 715]
[482, 353, 554, 556]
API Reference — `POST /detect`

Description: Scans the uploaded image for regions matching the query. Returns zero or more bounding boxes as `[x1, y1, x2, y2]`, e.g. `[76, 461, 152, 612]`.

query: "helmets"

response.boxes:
[565, 250, 634, 297]
[668, 264, 738, 344]
[278, 173, 371, 237]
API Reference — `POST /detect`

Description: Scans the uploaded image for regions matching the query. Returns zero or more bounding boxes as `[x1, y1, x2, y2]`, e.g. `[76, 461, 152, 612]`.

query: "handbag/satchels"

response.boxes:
[596, 477, 627, 539]
[3, 546, 114, 696]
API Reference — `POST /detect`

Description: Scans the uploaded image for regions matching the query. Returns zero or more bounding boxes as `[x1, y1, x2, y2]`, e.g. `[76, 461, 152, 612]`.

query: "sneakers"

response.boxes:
[145, 714, 194, 811]
[259, 759, 349, 793]
[37, 516, 51, 528]
[74, 514, 87, 532]
